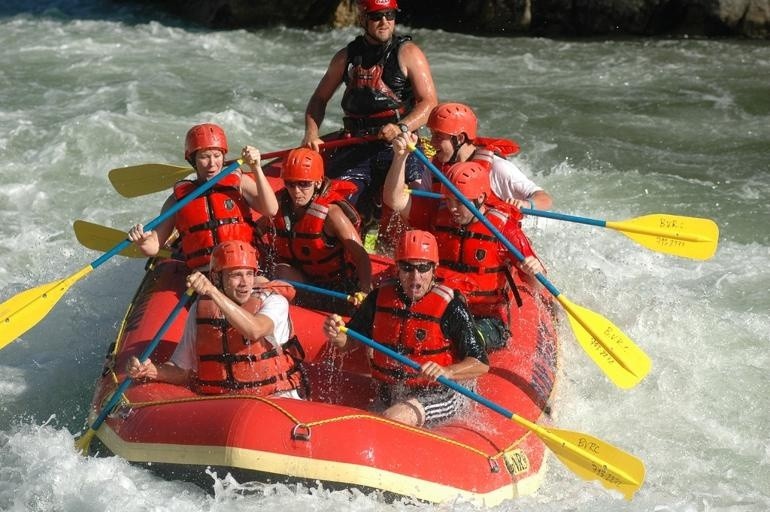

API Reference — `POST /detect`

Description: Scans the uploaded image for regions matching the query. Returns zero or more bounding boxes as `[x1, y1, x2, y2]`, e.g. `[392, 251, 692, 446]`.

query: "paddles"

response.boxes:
[406, 141, 653, 390]
[107, 134, 382, 199]
[0, 160, 245, 348]
[406, 190, 719, 260]
[338, 326, 645, 502]
[73, 219, 364, 307]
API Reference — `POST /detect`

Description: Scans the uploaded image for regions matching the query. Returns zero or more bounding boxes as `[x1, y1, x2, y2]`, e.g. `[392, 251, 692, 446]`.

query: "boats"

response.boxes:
[83, 152, 568, 509]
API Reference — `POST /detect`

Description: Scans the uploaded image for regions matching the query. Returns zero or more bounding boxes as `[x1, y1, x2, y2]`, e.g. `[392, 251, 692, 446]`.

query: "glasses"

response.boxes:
[285, 181, 312, 190]
[398, 260, 432, 273]
[368, 11, 394, 21]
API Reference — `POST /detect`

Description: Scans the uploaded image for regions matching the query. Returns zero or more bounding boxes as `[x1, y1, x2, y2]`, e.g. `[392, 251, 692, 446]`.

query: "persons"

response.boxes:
[319, 230, 492, 432]
[413, 100, 555, 226]
[299, 0, 441, 206]
[124, 237, 312, 408]
[127, 123, 282, 287]
[378, 131, 548, 352]
[260, 146, 374, 315]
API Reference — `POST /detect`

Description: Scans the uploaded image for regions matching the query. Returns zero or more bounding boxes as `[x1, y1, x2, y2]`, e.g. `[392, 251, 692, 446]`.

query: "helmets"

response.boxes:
[440, 162, 490, 201]
[426, 102, 478, 140]
[184, 123, 228, 160]
[280, 147, 325, 182]
[394, 229, 439, 264]
[358, 0, 397, 13]
[209, 241, 258, 285]
